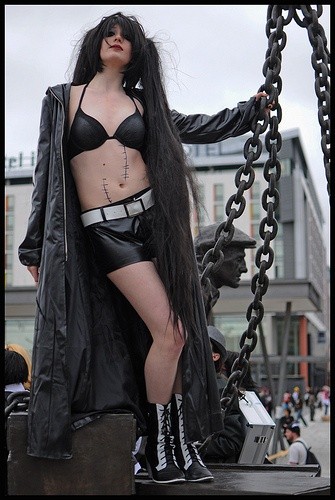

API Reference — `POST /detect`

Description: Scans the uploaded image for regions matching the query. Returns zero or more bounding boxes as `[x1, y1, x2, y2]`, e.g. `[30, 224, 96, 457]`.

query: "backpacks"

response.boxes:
[296, 440, 321, 477]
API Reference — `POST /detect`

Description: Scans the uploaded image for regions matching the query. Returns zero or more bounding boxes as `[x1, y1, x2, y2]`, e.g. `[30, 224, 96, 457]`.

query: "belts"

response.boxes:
[80, 189, 157, 227]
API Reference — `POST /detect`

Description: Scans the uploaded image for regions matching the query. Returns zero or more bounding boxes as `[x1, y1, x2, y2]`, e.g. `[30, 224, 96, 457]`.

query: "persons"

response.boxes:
[16, 11, 275, 484]
[195, 222, 257, 319]
[218, 351, 265, 407]
[4, 344, 32, 413]
[193, 326, 245, 463]
[258, 380, 330, 428]
[264, 407, 310, 466]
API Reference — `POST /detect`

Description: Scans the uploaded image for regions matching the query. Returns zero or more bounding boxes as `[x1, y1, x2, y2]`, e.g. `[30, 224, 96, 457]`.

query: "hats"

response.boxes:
[284, 423, 300, 435]
[207, 325, 228, 362]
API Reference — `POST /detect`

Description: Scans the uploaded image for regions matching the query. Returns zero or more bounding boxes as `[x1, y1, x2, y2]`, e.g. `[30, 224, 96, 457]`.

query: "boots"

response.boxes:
[170, 393, 214, 482]
[147, 400, 185, 483]
